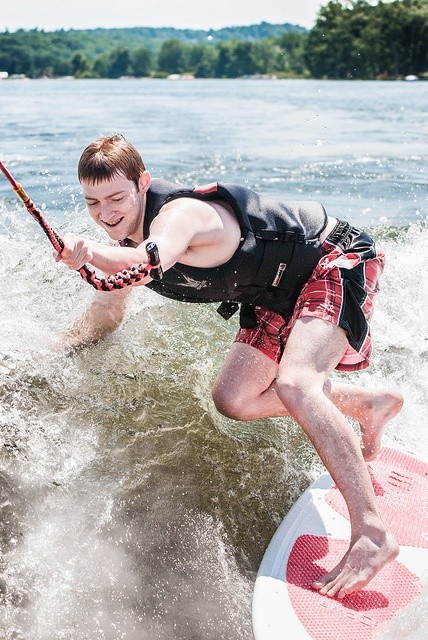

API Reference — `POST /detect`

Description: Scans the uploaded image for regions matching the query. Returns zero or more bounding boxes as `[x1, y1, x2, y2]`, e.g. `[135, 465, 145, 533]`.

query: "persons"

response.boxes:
[51, 134, 405, 598]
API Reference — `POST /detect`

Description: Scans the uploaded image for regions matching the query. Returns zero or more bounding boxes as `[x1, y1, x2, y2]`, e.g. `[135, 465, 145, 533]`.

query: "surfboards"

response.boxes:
[251, 404, 428, 640]
